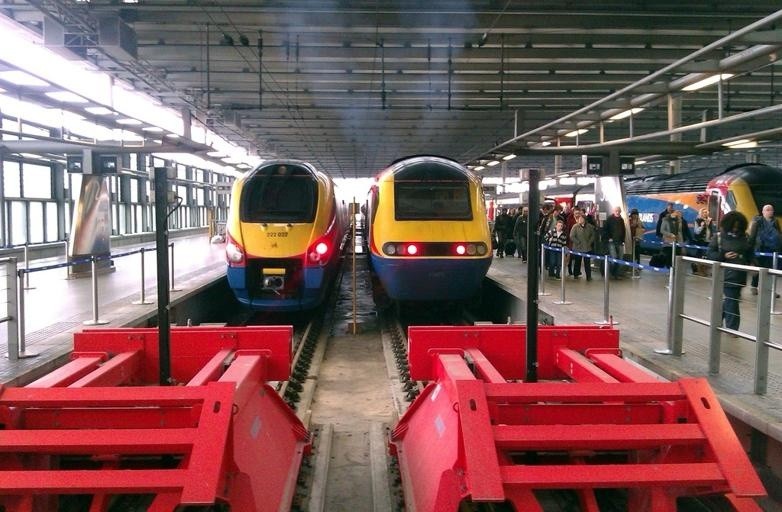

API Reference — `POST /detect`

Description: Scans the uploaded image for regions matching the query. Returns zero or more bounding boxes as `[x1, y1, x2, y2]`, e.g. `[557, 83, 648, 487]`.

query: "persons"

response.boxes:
[750, 205, 781, 298]
[490, 203, 717, 283]
[706, 210, 754, 338]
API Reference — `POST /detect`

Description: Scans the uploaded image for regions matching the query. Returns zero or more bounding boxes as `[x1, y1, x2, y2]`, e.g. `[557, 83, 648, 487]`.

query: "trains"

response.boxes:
[223, 158, 352, 314]
[360, 154, 493, 318]
[485, 161, 782, 267]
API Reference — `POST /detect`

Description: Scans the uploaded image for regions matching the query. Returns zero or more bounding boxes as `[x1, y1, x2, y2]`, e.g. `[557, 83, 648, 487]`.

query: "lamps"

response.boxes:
[469, 44, 782, 171]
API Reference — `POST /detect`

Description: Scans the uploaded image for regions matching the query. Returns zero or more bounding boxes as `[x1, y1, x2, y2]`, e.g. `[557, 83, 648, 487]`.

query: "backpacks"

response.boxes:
[755, 217, 782, 252]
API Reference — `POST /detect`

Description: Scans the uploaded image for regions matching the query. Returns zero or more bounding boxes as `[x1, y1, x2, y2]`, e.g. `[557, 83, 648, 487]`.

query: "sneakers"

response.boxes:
[638, 264, 644, 270]
[772, 292, 780, 298]
[751, 287, 757, 295]
[496, 254, 592, 282]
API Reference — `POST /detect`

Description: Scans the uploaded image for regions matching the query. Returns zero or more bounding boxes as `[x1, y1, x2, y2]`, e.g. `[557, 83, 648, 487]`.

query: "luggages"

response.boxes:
[649, 253, 666, 272]
[504, 237, 516, 258]
